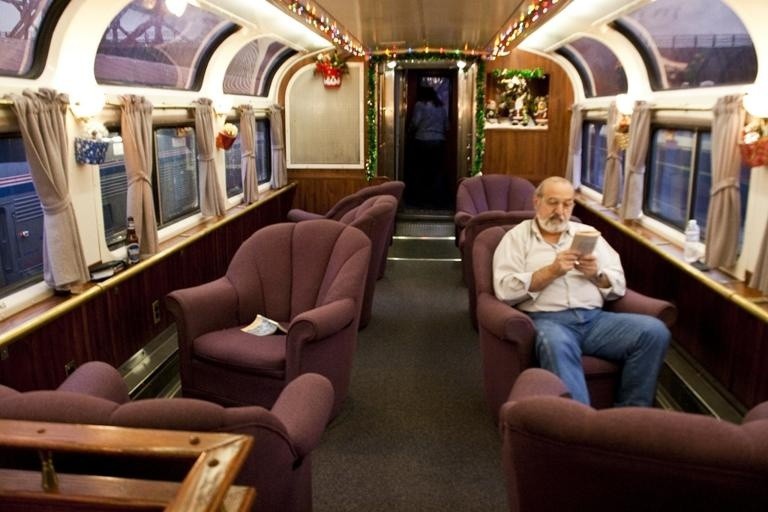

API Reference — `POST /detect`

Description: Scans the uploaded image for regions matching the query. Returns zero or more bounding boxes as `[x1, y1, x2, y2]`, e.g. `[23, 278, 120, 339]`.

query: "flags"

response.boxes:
[319, 65, 343, 88]
[218, 131, 237, 150]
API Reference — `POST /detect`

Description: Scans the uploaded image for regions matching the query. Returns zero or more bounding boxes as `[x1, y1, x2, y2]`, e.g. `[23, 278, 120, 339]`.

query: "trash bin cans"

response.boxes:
[589, 270, 604, 285]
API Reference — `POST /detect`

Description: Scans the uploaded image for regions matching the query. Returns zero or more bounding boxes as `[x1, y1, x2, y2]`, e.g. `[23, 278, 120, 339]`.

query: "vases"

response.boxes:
[218, 131, 237, 150]
[311, 50, 350, 78]
[319, 65, 343, 88]
[224, 122, 238, 139]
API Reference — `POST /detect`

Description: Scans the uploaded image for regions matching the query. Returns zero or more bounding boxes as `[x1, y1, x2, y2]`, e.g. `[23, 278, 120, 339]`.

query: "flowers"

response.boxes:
[311, 50, 350, 78]
[224, 122, 238, 139]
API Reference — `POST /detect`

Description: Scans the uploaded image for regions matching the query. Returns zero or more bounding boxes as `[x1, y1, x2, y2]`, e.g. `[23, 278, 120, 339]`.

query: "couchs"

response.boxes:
[325, 180, 406, 248]
[162, 218, 372, 424]
[498, 367, 766, 511]
[455, 175, 537, 245]
[289, 195, 399, 331]
[473, 223, 677, 421]
[461, 209, 536, 265]
[0, 361, 335, 510]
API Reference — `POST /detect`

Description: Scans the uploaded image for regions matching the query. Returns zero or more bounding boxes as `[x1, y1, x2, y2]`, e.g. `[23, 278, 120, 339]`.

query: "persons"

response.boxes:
[491, 176, 673, 411]
[407, 84, 449, 210]
[504, 91, 537, 127]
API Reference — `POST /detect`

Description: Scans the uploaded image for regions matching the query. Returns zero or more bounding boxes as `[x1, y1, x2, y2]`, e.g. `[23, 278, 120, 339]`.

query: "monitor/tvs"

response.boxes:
[569, 230, 602, 261]
[239, 313, 289, 337]
[89, 259, 125, 281]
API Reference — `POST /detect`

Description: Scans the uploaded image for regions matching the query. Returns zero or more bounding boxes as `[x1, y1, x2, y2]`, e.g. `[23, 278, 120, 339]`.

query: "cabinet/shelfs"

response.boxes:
[125, 217, 140, 263]
[685, 219, 701, 263]
